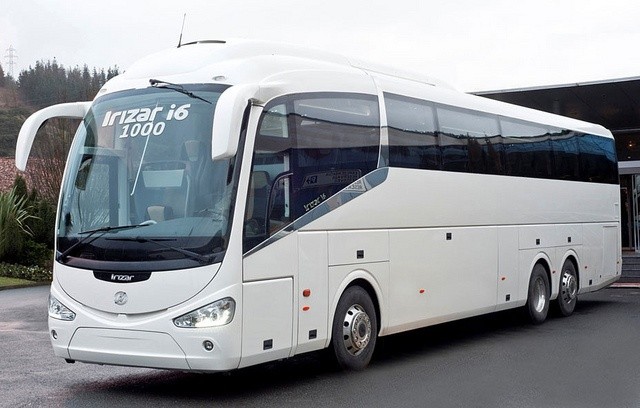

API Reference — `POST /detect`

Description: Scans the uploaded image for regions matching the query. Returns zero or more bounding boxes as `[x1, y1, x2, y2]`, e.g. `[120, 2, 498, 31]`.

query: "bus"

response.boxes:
[14, 12, 624, 376]
